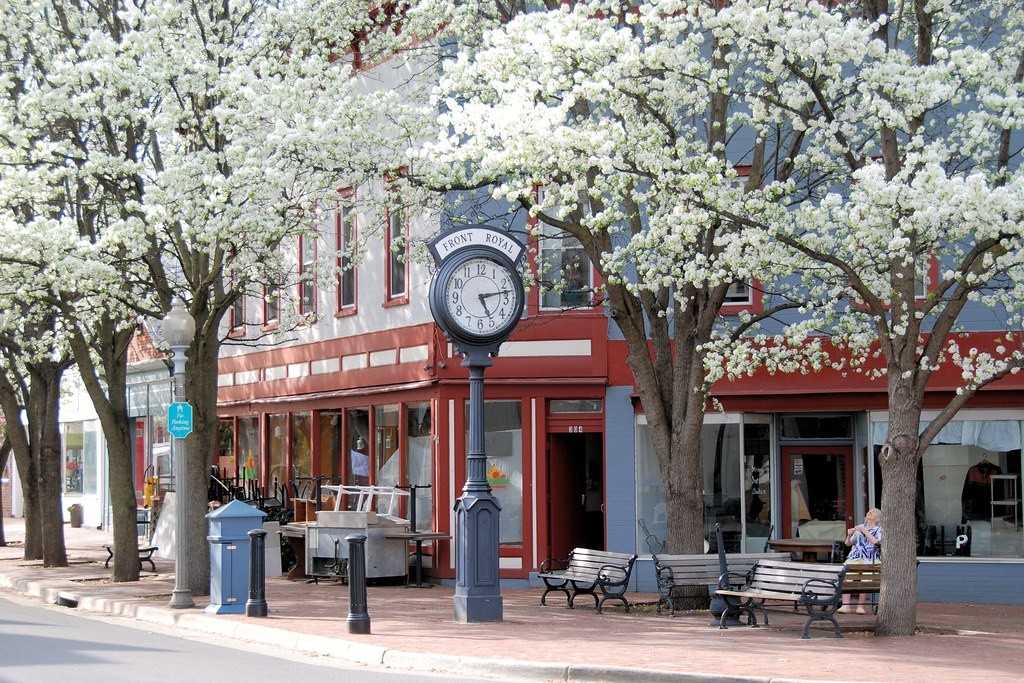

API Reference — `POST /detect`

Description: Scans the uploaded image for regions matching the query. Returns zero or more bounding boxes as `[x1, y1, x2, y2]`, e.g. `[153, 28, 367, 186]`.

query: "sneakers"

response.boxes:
[856, 607, 865, 614]
[837, 607, 851, 614]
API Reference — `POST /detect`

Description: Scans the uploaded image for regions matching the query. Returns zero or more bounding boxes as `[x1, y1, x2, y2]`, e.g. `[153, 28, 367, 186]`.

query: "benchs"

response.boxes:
[715, 557, 846, 640]
[537, 547, 638, 614]
[102, 544, 159, 572]
[791, 561, 920, 611]
[651, 552, 764, 616]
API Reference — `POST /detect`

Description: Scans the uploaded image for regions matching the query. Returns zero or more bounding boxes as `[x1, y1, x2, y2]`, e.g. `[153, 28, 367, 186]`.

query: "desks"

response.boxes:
[384, 536, 452, 588]
[766, 538, 839, 562]
[798, 520, 845, 541]
[384, 484, 447, 536]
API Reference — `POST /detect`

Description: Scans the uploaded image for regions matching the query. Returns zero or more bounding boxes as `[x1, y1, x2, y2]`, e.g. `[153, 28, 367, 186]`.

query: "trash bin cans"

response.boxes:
[202, 498, 272, 615]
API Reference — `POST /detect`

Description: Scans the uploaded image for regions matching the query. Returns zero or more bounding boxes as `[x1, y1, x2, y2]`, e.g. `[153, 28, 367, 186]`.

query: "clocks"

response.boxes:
[444, 258, 519, 336]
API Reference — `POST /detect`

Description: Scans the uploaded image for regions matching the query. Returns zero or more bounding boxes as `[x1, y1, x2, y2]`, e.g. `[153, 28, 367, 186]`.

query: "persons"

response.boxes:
[838, 508, 882, 615]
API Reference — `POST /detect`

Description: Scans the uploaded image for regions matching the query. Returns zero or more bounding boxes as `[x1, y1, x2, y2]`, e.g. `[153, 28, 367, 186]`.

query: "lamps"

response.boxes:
[385, 428, 393, 448]
[356, 437, 366, 450]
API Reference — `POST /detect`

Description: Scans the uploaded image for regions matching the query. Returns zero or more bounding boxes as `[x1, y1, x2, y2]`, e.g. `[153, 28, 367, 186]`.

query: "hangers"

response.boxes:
[981, 452, 991, 465]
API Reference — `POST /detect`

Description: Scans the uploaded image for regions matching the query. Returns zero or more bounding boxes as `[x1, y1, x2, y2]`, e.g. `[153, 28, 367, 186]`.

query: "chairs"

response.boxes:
[213, 465, 305, 524]
